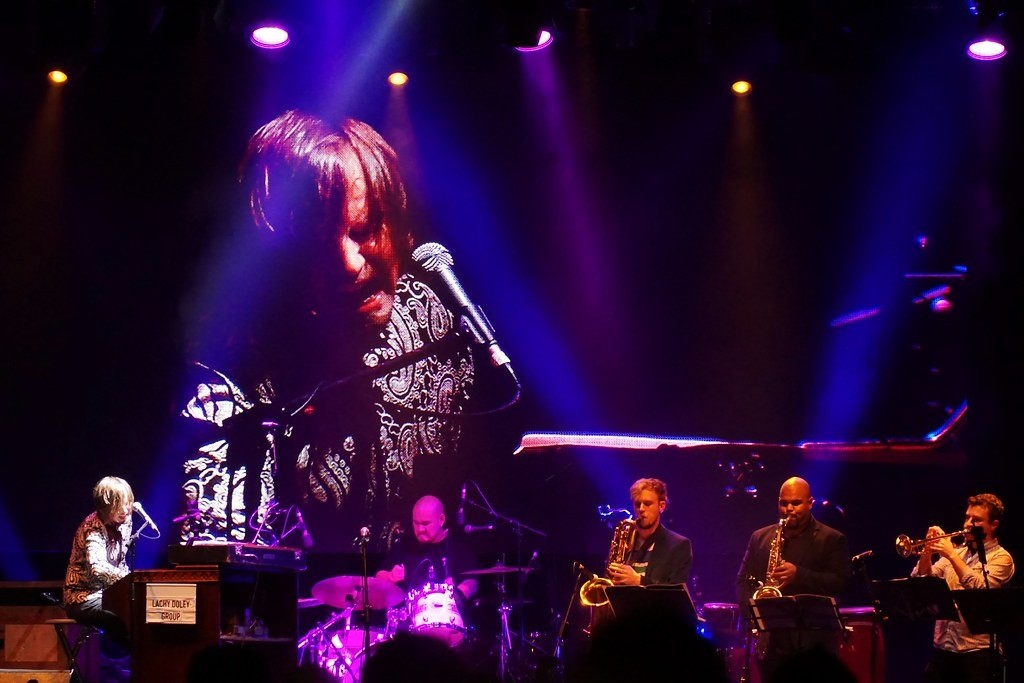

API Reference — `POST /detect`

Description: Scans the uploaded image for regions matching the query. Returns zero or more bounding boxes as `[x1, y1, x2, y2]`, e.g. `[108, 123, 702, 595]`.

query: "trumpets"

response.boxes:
[895, 528, 972, 558]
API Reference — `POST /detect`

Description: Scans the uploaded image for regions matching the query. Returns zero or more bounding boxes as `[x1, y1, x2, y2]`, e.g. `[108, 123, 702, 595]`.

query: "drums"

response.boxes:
[498, 632, 565, 683]
[398, 584, 468, 647]
[307, 625, 383, 677]
[341, 639, 394, 683]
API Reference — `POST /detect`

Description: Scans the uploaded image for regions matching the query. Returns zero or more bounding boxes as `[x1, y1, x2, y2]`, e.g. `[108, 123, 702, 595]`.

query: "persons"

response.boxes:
[608, 478, 693, 586]
[63, 477, 134, 629]
[734, 477, 852, 683]
[910, 494, 1016, 683]
[373, 494, 478, 598]
[182, 109, 486, 554]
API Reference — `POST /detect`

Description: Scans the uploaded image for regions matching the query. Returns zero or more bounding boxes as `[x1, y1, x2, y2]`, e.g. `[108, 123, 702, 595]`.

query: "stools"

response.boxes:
[45, 619, 88, 683]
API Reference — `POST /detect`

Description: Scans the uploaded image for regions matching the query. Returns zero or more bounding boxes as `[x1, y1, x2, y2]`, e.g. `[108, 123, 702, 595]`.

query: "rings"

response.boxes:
[621, 569, 622, 572]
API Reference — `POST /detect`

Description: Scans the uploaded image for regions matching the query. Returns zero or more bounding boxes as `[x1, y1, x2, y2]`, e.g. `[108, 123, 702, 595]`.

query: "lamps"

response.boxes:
[47, 52, 74, 84]
[500, 0, 555, 53]
[968, 0, 1007, 61]
[250, 0, 291, 50]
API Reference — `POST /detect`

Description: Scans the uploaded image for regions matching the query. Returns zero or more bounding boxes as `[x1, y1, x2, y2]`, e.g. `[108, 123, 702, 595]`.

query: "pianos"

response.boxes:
[102, 568, 300, 683]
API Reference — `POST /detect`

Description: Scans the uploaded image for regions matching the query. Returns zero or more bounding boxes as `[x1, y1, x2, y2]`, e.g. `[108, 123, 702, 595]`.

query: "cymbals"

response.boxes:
[474, 596, 531, 604]
[311, 575, 406, 609]
[461, 565, 534, 574]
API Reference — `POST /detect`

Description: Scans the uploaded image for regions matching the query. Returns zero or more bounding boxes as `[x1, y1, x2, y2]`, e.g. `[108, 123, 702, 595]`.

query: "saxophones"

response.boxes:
[752, 515, 790, 660]
[578, 517, 641, 637]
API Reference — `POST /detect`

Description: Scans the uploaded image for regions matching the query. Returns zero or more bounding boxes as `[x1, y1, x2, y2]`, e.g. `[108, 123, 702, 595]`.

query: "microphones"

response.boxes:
[457, 481, 467, 525]
[294, 505, 313, 547]
[411, 242, 514, 382]
[747, 574, 763, 590]
[852, 550, 873, 562]
[173, 514, 191, 523]
[360, 526, 369, 539]
[132, 502, 157, 532]
[574, 561, 598, 581]
[464, 524, 495, 533]
